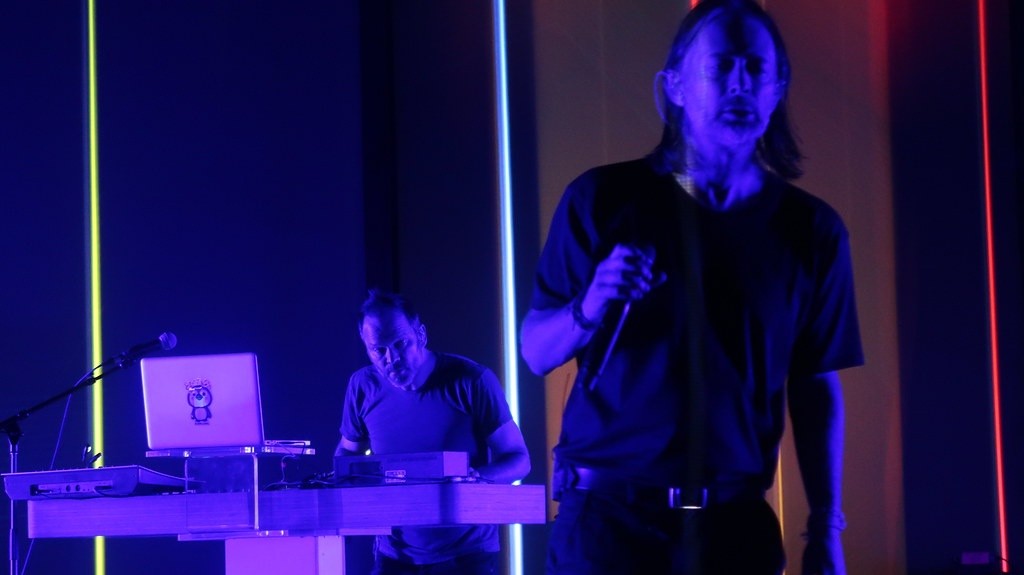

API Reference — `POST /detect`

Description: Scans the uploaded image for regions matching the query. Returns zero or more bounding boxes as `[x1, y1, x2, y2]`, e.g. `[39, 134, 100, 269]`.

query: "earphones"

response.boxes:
[669, 78, 682, 95]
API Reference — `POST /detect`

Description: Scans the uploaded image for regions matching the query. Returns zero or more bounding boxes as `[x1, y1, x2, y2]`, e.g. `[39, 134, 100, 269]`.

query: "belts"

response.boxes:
[562, 464, 767, 514]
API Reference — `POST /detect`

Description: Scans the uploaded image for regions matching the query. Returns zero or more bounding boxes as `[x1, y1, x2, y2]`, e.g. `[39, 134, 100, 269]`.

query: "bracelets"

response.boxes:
[571, 287, 602, 332]
[806, 508, 847, 530]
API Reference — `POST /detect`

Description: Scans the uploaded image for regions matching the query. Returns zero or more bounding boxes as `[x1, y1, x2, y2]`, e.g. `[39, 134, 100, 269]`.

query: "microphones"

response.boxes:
[580, 246, 656, 392]
[106, 333, 177, 364]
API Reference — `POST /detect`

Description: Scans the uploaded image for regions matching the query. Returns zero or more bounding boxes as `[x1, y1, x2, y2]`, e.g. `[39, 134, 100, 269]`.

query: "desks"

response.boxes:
[27, 483, 546, 575]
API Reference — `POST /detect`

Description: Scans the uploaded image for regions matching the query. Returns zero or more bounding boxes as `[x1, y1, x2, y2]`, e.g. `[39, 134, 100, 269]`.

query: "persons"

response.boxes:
[334, 290, 531, 575]
[519, 0, 866, 575]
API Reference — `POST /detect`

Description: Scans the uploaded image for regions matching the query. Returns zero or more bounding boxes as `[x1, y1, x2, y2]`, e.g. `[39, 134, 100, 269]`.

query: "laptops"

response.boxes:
[138, 353, 311, 448]
